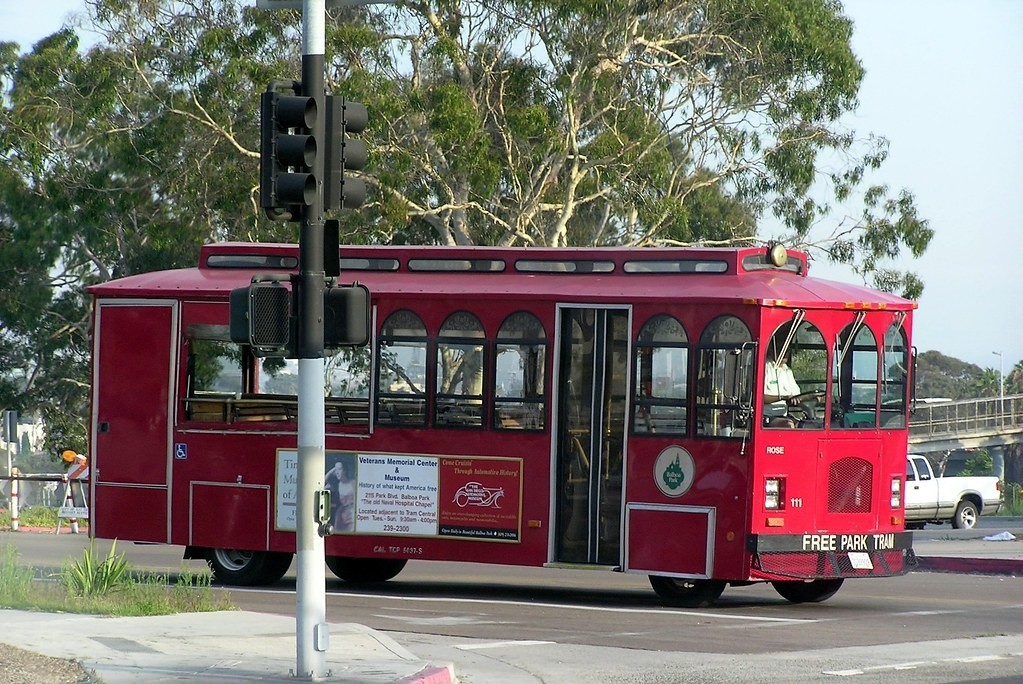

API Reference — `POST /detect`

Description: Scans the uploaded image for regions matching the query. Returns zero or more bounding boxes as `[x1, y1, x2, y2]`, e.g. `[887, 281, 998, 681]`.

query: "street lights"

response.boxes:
[866, 397, 952, 410]
[993, 350, 1005, 430]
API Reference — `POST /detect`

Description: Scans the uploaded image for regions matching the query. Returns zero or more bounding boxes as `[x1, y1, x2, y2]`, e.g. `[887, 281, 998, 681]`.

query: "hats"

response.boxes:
[62, 450, 76, 462]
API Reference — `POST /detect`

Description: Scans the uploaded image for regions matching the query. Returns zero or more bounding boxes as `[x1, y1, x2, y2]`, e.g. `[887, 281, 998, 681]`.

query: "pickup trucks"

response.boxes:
[903, 452, 1003, 534]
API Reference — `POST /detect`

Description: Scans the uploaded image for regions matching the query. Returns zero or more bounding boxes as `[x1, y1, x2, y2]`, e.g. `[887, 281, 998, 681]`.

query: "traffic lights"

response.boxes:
[259, 90, 318, 223]
[326, 88, 369, 210]
[229, 284, 289, 348]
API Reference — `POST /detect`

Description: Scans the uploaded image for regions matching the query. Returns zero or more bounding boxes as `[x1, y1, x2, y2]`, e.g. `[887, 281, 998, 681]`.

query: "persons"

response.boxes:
[324, 459, 355, 532]
[743, 335, 820, 428]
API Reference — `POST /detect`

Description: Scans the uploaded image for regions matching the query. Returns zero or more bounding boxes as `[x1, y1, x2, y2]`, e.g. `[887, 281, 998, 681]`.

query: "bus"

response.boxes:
[89, 239, 918, 614]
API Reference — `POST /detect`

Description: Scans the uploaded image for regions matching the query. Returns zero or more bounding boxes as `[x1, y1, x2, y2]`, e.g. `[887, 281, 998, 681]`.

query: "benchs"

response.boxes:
[189, 390, 706, 437]
